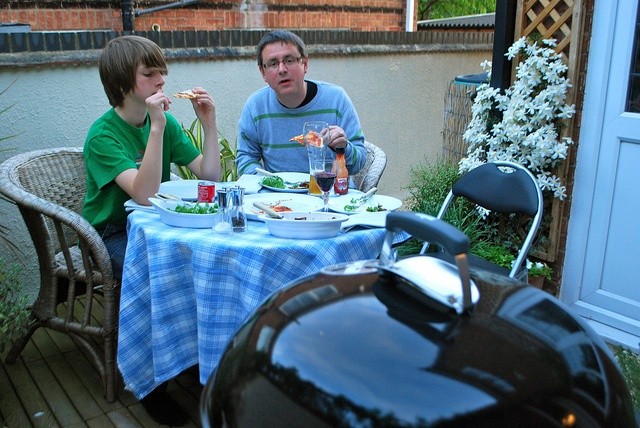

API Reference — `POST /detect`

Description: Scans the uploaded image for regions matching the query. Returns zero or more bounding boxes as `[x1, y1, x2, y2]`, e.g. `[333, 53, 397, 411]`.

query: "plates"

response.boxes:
[244, 190, 325, 220]
[329, 195, 402, 214]
[159, 178, 224, 202]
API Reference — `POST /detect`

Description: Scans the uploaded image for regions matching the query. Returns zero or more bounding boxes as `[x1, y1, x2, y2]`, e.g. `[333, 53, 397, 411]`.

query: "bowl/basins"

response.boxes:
[258, 211, 348, 239]
[150, 198, 232, 229]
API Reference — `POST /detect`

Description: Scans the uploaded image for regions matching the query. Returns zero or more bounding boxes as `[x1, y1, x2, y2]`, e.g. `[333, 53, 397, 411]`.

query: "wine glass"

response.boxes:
[313, 160, 338, 212]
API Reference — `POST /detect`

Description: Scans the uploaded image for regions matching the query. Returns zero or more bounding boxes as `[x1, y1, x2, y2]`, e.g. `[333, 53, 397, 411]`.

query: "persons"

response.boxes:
[235, 31, 367, 176]
[78, 36, 215, 426]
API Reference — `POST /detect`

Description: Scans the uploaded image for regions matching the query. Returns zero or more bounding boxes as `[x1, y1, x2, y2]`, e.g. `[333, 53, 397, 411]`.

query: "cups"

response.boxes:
[304, 120, 330, 195]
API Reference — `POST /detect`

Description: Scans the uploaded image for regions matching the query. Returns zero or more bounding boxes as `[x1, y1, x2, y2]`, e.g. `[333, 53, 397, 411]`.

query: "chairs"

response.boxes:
[350, 141, 387, 195]
[0, 145, 118, 403]
[419, 160, 544, 287]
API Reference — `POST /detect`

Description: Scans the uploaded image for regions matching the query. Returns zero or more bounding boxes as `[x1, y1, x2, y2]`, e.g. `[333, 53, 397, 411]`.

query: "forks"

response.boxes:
[255, 167, 301, 185]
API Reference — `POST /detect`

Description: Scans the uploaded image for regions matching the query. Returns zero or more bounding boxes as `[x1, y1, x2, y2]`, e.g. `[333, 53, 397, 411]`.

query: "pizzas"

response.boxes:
[173, 89, 198, 99]
[288, 130, 323, 148]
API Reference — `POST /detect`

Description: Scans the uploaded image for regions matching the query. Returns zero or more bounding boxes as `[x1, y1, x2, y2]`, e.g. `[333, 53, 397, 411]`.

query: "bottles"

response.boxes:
[334, 146, 349, 195]
[213, 189, 233, 233]
[231, 185, 248, 234]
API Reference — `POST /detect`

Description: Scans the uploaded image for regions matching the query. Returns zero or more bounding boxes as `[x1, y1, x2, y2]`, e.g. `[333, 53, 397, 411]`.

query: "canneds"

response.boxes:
[198, 181, 215, 202]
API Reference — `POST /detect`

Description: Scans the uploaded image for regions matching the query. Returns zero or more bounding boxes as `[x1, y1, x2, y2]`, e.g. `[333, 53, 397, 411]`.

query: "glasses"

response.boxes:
[262, 54, 303, 69]
